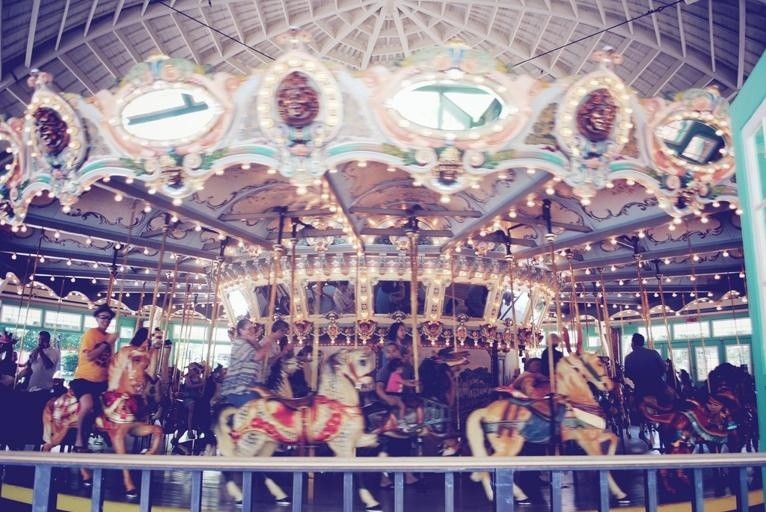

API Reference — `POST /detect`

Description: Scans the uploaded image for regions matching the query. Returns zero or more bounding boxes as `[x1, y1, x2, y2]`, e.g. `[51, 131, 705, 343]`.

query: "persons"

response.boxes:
[1, 298, 682, 454]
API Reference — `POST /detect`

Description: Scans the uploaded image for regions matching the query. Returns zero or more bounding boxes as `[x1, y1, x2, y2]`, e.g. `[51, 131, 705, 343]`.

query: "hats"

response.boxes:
[93, 303, 115, 318]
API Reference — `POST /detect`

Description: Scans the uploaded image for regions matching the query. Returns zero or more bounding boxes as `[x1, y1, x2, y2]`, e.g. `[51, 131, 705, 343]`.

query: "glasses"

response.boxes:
[95, 315, 112, 320]
